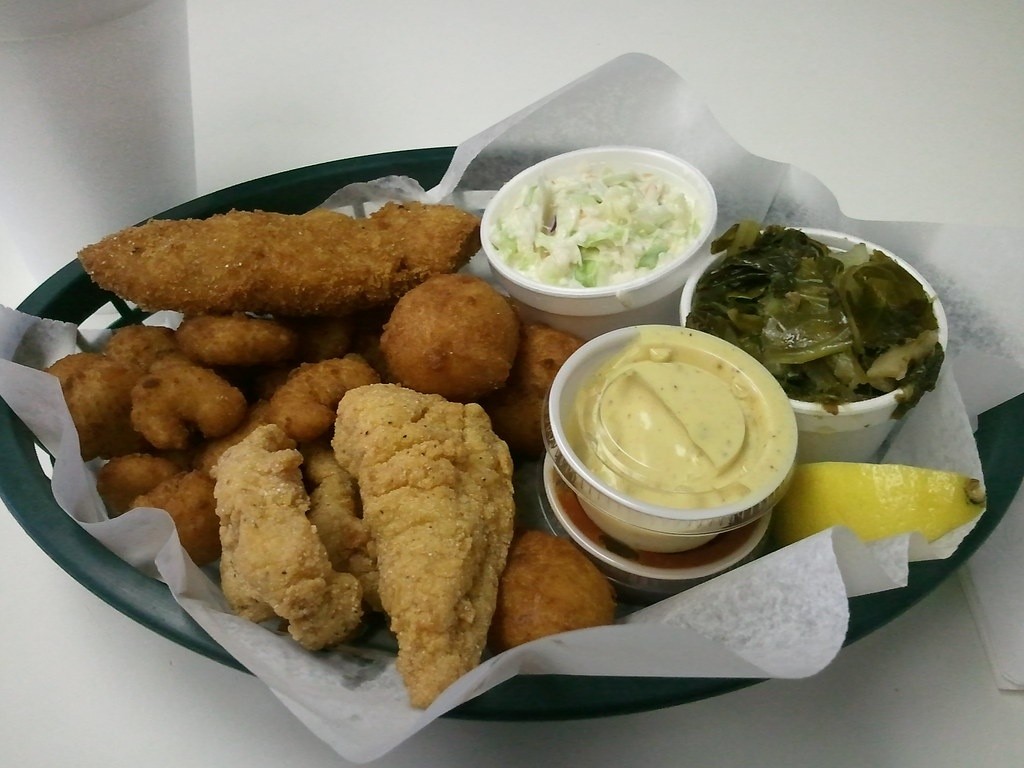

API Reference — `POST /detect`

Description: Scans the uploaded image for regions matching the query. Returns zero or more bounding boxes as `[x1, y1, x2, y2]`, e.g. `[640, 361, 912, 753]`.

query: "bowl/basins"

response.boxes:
[540, 324, 797, 555]
[480, 144, 718, 340]
[680, 228, 949, 465]
[544, 447, 772, 603]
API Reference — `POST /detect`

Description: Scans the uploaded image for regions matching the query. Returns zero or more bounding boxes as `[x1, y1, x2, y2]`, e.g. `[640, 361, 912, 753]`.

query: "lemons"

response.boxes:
[774, 462, 989, 545]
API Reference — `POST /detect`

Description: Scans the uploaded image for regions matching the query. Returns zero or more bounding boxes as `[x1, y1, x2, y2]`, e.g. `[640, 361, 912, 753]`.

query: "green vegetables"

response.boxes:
[685, 218, 945, 419]
